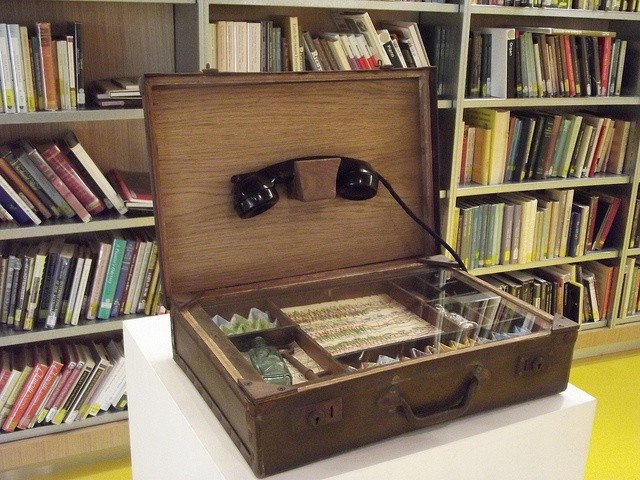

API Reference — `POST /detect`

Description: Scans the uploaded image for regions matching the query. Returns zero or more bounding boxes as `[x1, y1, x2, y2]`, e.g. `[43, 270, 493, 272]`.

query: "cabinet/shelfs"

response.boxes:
[0, 2, 640, 479]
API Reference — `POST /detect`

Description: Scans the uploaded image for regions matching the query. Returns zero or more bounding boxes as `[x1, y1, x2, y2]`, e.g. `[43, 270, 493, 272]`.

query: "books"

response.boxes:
[459, 106, 639, 186]
[0, 129, 157, 223]
[1, 336, 126, 433]
[204, 12, 458, 96]
[0, 19, 145, 113]
[0, 227, 170, 331]
[480, 260, 640, 325]
[456, 188, 638, 271]
[466, 2, 640, 100]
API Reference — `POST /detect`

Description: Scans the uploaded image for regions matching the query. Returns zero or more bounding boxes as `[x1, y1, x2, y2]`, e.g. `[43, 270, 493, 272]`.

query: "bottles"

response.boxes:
[248, 336, 292, 387]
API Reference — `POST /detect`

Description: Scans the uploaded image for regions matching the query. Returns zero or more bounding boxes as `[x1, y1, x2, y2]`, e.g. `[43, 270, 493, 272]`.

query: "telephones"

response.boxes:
[229, 155, 378, 218]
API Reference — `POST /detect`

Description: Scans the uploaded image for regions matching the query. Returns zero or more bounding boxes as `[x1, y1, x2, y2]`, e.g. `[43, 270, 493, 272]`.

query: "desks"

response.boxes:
[120, 314, 593, 480]
